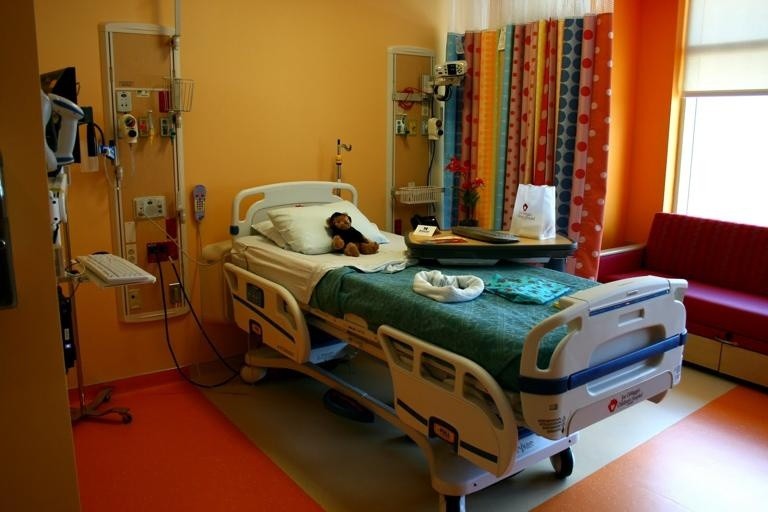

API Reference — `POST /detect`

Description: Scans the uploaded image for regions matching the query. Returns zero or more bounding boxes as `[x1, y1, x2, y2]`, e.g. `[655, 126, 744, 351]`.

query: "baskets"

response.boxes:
[160, 76, 197, 112]
[398, 185, 442, 205]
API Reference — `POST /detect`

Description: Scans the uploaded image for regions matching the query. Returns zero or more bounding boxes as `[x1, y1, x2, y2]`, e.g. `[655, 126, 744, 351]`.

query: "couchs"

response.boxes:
[596, 211, 768, 389]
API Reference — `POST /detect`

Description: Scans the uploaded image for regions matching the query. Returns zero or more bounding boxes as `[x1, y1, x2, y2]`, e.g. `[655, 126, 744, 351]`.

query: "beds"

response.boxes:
[222, 181, 689, 512]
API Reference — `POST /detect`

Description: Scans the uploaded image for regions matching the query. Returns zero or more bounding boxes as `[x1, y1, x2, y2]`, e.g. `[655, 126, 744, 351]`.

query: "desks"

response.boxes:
[405, 230, 577, 272]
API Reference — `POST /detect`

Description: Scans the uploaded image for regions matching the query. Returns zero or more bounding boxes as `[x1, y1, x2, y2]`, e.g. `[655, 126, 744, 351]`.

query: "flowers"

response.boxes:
[444, 156, 484, 221]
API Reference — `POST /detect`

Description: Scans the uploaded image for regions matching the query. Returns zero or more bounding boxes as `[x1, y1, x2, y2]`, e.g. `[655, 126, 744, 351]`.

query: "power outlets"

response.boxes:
[147, 243, 177, 261]
[135, 196, 166, 218]
[117, 91, 132, 113]
[126, 245, 137, 264]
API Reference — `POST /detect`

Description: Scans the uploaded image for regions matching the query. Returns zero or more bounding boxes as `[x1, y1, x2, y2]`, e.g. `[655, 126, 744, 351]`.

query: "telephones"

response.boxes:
[411, 215, 440, 231]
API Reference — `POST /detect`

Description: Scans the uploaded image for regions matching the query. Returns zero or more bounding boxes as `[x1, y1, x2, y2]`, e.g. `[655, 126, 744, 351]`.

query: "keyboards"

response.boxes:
[77, 254, 148, 283]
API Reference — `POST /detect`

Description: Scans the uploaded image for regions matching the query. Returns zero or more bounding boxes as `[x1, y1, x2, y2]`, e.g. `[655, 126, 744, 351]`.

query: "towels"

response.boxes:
[411, 270, 486, 302]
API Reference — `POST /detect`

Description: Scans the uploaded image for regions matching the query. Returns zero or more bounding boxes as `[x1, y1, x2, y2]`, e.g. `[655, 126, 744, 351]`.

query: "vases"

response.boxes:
[460, 220, 478, 226]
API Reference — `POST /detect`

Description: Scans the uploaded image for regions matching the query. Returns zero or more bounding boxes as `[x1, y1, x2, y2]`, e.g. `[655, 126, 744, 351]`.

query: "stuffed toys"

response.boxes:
[327, 211, 380, 257]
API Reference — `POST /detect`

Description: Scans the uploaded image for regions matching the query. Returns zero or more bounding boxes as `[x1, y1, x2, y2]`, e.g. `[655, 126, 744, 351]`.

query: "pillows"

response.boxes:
[268, 200, 389, 255]
[250, 220, 291, 250]
